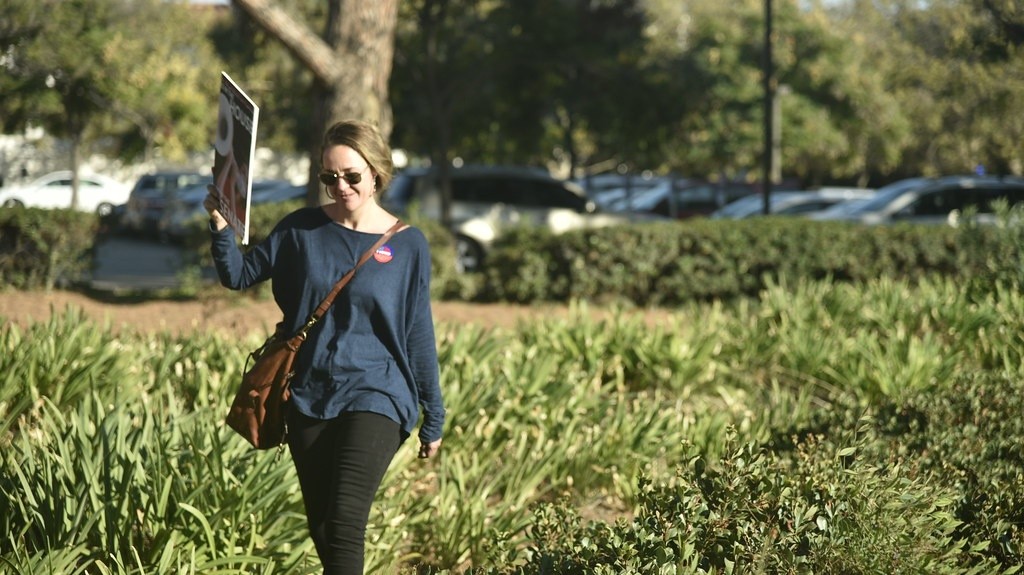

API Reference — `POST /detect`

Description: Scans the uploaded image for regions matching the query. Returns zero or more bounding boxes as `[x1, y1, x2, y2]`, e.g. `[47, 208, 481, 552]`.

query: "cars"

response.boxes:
[382, 168, 596, 276]
[88, 170, 215, 237]
[252, 178, 306, 211]
[579, 172, 1024, 225]
[1, 170, 132, 219]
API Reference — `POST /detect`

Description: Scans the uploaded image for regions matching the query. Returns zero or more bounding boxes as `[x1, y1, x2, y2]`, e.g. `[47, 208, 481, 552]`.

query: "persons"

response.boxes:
[205, 117, 445, 575]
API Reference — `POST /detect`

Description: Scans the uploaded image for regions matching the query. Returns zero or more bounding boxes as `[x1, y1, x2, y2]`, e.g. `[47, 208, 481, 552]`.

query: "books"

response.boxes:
[211, 71, 260, 246]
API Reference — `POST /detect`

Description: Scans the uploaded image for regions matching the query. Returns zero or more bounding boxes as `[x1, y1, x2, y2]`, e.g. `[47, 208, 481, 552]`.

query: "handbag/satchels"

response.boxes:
[225, 322, 307, 450]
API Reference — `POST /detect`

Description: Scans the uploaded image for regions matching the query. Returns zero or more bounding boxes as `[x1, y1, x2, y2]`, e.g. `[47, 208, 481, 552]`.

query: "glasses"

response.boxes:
[318, 163, 372, 185]
